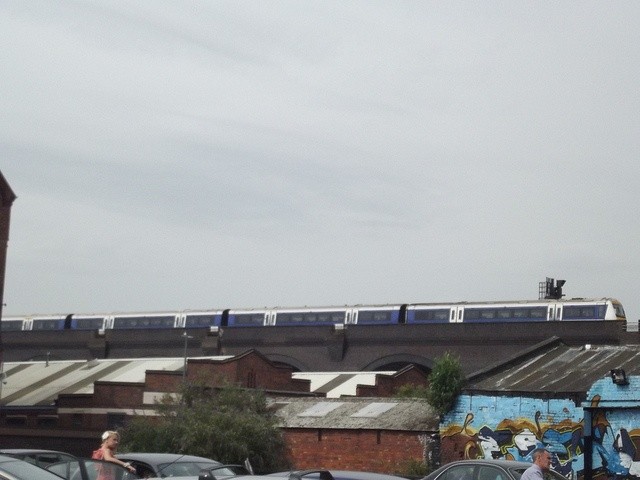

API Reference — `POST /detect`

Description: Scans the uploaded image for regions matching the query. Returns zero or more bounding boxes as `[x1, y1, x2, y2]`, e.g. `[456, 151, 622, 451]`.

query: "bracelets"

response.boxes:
[123, 462, 130, 467]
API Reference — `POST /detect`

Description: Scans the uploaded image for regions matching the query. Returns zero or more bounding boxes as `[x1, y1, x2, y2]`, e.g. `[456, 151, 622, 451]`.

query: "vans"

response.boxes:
[1, 449, 79, 469]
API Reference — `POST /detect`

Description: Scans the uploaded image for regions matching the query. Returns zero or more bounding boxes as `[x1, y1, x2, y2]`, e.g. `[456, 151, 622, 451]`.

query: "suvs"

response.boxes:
[199, 463, 407, 480]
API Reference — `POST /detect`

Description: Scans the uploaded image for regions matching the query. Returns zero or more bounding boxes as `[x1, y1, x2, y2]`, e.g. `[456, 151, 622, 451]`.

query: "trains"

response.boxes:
[0, 297, 628, 334]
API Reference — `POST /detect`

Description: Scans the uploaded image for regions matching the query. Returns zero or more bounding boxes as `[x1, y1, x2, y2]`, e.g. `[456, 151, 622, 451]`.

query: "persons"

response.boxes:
[89, 429, 137, 480]
[519, 448, 553, 480]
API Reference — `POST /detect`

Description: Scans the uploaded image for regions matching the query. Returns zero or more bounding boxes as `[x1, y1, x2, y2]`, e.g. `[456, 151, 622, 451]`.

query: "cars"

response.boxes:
[0, 454, 68, 480]
[44, 451, 237, 480]
[419, 459, 568, 480]
